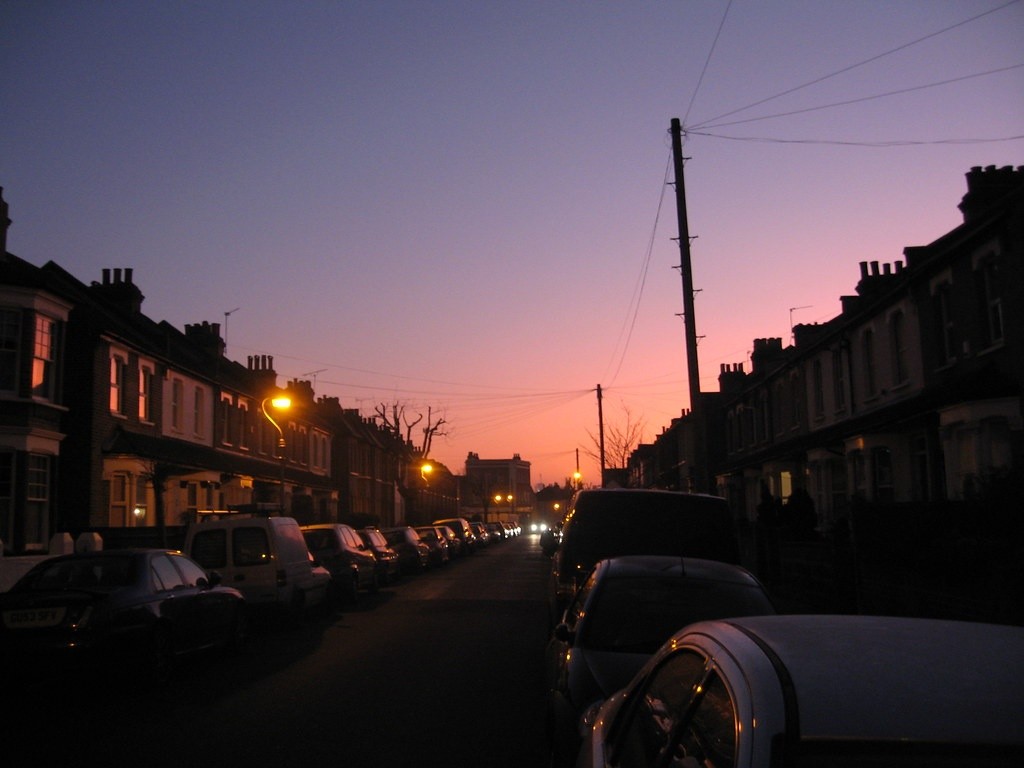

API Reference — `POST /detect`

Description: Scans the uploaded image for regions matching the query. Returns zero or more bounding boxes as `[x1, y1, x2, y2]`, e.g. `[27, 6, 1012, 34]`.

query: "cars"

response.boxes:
[431, 517, 522, 561]
[383, 527, 431, 576]
[1, 547, 261, 711]
[413, 526, 448, 568]
[357, 527, 401, 585]
[635, 693, 700, 768]
[530, 520, 550, 535]
[571, 611, 1024, 768]
[541, 555, 781, 768]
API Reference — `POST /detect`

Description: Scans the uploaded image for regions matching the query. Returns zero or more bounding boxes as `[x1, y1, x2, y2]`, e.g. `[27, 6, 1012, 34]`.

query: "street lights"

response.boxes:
[505, 494, 514, 521]
[260, 392, 290, 515]
[420, 464, 433, 526]
[493, 493, 504, 521]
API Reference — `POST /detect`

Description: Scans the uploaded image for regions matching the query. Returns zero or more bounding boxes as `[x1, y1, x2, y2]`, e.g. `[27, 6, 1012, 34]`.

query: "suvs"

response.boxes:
[552, 486, 745, 637]
[300, 522, 380, 604]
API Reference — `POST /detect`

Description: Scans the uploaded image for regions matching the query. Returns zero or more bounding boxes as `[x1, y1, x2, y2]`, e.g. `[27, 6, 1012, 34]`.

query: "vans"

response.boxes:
[181, 502, 333, 625]
